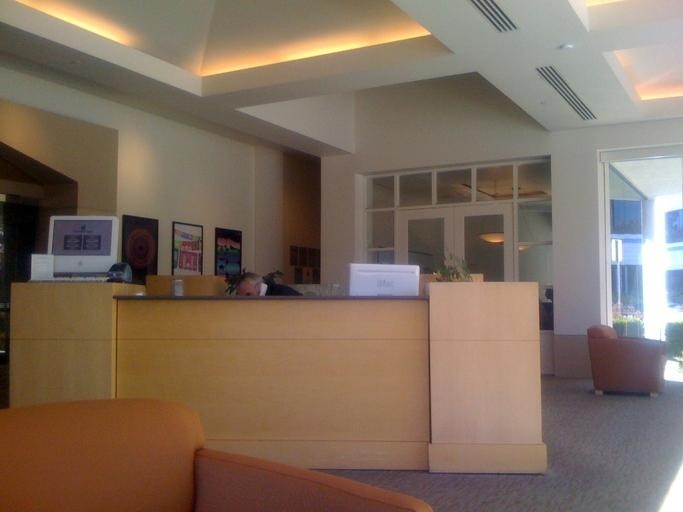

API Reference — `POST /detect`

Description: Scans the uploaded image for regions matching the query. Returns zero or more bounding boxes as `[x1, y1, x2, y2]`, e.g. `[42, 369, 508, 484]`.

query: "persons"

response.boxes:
[235, 272, 303, 297]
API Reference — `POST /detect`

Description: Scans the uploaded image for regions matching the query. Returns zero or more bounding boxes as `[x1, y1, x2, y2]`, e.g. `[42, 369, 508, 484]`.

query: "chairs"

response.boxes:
[0, 395, 438, 512]
[586, 322, 668, 402]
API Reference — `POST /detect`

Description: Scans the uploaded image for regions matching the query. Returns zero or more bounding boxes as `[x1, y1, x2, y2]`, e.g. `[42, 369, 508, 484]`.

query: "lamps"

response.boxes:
[516, 209, 534, 253]
[476, 212, 503, 244]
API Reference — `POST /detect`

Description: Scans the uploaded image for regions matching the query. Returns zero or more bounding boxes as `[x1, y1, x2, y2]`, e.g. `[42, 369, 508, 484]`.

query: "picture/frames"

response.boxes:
[120, 213, 160, 279]
[169, 220, 203, 275]
[212, 226, 242, 276]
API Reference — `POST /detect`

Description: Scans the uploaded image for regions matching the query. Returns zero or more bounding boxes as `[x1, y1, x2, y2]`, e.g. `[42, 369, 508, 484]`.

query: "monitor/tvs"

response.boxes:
[47, 215, 119, 281]
[349, 263, 420, 297]
